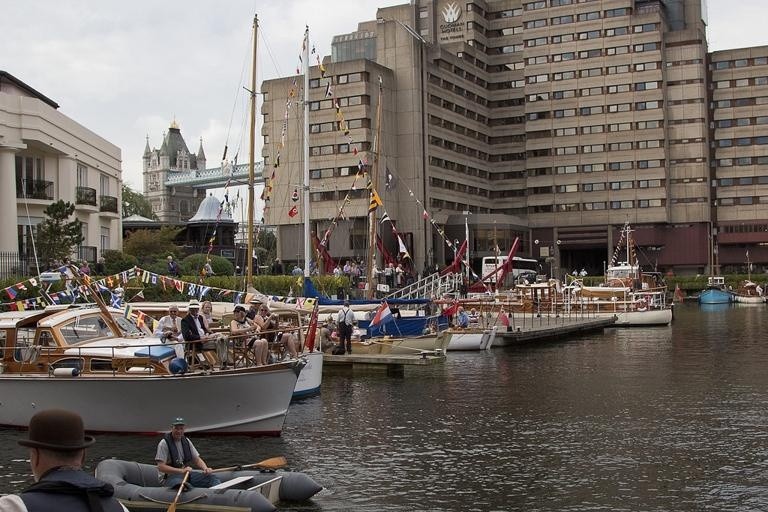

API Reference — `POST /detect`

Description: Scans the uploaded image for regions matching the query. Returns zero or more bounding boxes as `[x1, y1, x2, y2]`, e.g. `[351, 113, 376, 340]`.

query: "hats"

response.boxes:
[16, 408, 97, 451]
[171, 416, 186, 426]
[188, 299, 201, 308]
[233, 306, 245, 313]
[249, 296, 263, 304]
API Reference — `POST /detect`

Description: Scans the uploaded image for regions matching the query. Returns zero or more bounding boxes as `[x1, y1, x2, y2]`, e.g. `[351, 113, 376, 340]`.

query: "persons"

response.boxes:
[318, 301, 356, 354]
[423, 306, 478, 334]
[153, 415, 221, 490]
[166, 256, 440, 288]
[579, 268, 588, 277]
[728, 284, 733, 292]
[756, 284, 763, 296]
[0, 408, 130, 512]
[153, 299, 297, 368]
[571, 269, 578, 277]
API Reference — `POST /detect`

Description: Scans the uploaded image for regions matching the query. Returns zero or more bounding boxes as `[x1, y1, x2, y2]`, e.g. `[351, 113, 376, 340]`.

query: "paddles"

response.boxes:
[167, 470, 190, 512]
[202, 456, 286, 473]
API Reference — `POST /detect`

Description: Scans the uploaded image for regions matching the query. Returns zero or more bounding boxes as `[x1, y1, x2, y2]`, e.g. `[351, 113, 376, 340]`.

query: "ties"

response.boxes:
[172, 319, 177, 332]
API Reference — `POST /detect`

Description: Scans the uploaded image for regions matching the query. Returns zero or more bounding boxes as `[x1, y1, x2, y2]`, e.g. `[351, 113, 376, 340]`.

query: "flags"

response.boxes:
[369, 302, 393, 331]
[285, 188, 299, 220]
[443, 304, 458, 314]
[462, 260, 478, 277]
[305, 301, 318, 353]
[160, 276, 316, 310]
[0, 278, 61, 312]
[61, 266, 159, 331]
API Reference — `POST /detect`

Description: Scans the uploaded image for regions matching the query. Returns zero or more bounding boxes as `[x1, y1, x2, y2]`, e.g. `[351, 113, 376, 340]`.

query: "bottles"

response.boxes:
[220, 316, 225, 329]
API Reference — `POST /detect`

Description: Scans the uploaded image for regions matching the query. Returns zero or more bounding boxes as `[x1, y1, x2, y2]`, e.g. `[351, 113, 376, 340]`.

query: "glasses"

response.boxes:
[233, 310, 240, 313]
[169, 311, 178, 313]
[261, 309, 267, 312]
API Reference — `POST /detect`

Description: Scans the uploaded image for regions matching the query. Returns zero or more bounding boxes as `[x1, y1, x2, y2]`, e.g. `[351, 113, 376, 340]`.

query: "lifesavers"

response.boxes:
[635, 297, 649, 311]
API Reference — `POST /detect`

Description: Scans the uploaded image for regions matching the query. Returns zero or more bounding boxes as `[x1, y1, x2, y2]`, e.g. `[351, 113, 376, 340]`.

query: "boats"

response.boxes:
[94, 456, 326, 512]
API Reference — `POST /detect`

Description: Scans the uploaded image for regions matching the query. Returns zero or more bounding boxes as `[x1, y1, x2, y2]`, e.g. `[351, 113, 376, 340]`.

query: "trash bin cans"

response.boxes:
[337, 287, 345, 300]
